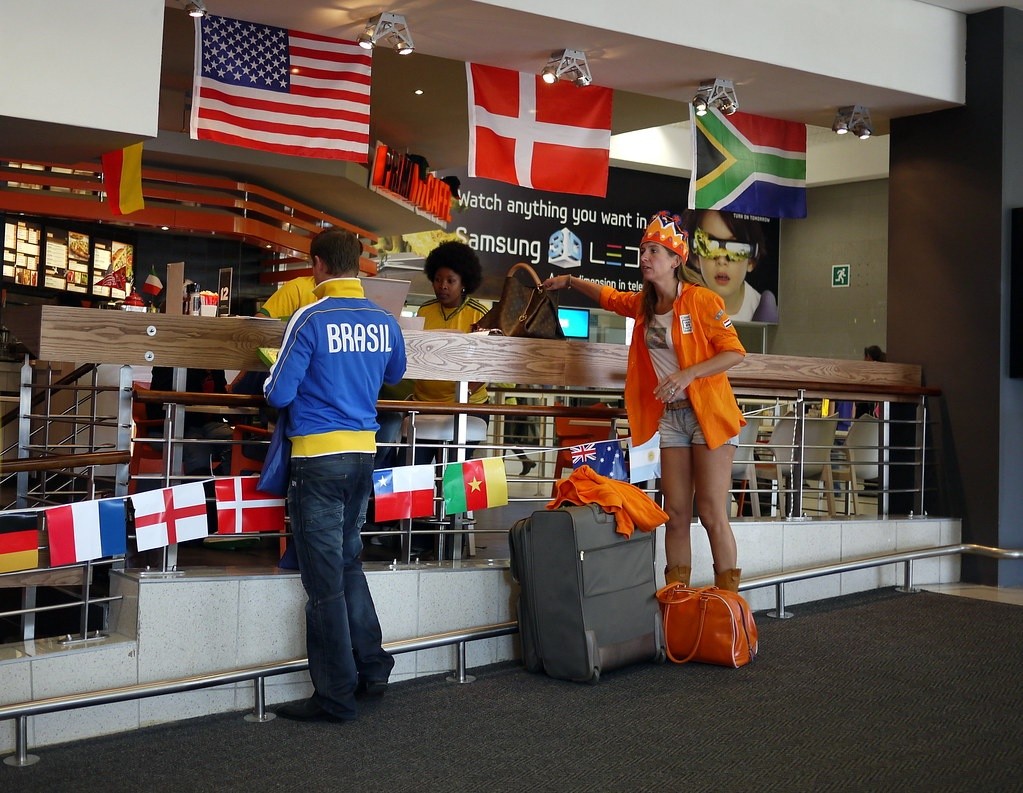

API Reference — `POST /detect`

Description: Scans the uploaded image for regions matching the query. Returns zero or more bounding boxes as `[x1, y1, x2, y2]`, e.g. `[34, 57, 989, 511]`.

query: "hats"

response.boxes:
[639, 211, 688, 265]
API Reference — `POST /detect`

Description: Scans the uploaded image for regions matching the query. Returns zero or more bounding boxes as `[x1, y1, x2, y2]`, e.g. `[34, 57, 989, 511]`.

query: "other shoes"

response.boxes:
[370, 527, 399, 545]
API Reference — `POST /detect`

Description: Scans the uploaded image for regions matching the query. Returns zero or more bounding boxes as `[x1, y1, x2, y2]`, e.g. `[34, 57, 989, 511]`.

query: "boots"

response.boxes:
[714, 564, 741, 592]
[665, 564, 692, 589]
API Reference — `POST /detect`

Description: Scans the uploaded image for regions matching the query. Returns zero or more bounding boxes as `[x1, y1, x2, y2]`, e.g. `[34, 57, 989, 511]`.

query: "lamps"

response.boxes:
[357, 13, 416, 54]
[832, 105, 872, 140]
[541, 49, 592, 87]
[181, 0, 208, 19]
[692, 78, 739, 117]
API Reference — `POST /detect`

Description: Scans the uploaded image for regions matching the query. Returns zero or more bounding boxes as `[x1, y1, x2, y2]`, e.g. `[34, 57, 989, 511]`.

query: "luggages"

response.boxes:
[509, 501, 667, 684]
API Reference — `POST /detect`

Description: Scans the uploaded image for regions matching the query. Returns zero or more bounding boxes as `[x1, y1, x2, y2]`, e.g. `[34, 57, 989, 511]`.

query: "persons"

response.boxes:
[145, 240, 539, 555]
[264, 227, 408, 723]
[549, 210, 746, 592]
[824, 345, 885, 497]
[682, 209, 779, 324]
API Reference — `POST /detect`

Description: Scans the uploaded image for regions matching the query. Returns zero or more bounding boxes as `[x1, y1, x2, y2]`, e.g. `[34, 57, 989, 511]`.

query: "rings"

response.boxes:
[670, 389, 674, 394]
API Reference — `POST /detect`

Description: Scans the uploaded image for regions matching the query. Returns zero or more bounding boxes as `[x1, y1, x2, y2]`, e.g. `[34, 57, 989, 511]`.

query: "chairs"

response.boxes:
[129, 380, 225, 510]
[229, 425, 287, 559]
[553, 401, 623, 498]
[741, 411, 840, 519]
[730, 408, 765, 519]
[821, 414, 882, 515]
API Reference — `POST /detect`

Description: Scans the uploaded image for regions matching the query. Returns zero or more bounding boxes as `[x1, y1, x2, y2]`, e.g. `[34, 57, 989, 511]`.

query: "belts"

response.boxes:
[666, 399, 692, 409]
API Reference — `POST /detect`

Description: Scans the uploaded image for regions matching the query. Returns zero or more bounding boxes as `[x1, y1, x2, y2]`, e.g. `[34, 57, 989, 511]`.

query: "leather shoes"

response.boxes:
[274, 698, 358, 723]
[355, 674, 386, 700]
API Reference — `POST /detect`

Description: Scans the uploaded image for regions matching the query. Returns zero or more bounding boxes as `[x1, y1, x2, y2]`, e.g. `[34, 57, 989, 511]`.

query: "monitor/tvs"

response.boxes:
[558, 306, 591, 342]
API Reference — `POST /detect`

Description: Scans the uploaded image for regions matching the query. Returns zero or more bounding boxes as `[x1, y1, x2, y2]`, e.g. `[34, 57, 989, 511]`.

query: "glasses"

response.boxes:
[693, 228, 754, 262]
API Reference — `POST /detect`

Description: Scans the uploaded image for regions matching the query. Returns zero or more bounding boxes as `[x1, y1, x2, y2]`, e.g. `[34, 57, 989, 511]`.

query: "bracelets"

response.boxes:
[567, 274, 572, 289]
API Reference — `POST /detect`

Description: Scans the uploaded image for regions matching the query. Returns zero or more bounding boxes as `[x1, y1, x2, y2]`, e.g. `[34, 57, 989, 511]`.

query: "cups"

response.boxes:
[200, 305, 218, 317]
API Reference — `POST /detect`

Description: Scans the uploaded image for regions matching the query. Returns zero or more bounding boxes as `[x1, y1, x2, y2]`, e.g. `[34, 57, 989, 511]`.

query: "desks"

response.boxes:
[161, 405, 260, 417]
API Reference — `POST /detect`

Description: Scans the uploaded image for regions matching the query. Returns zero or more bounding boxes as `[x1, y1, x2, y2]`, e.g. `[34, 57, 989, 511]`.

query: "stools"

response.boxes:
[403, 414, 487, 558]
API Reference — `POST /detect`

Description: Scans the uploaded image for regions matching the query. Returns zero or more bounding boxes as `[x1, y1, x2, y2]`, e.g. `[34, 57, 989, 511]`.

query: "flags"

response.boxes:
[442, 458, 509, 515]
[189, 13, 375, 163]
[626, 431, 662, 485]
[214, 477, 284, 533]
[102, 141, 144, 215]
[373, 465, 434, 522]
[465, 63, 612, 197]
[143, 274, 163, 295]
[569, 440, 628, 481]
[0, 512, 40, 573]
[131, 483, 210, 552]
[688, 103, 809, 219]
[45, 498, 128, 567]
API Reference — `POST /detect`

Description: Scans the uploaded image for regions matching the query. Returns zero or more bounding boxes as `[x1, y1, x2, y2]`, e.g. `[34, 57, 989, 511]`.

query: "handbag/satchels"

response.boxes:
[657, 582, 757, 667]
[255, 409, 293, 497]
[498, 262, 565, 339]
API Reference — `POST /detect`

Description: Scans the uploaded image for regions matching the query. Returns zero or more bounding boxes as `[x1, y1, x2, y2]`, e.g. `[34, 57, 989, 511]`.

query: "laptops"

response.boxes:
[357, 276, 412, 321]
